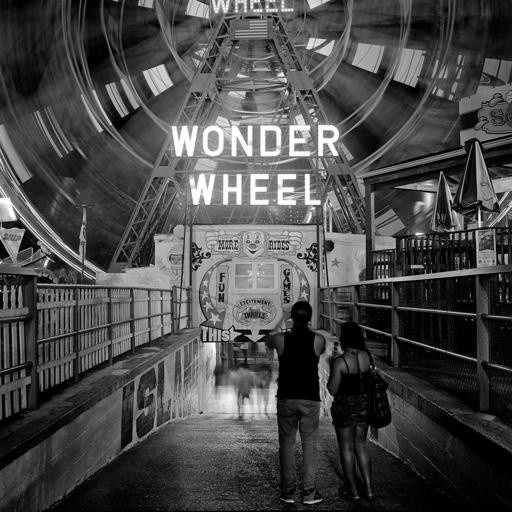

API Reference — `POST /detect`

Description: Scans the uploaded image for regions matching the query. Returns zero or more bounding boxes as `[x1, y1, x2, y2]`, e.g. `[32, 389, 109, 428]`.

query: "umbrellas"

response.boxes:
[429, 171, 462, 235]
[451, 139, 500, 228]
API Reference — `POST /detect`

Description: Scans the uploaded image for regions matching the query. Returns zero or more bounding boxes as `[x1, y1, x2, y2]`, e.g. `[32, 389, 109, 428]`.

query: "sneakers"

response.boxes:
[279, 487, 324, 504]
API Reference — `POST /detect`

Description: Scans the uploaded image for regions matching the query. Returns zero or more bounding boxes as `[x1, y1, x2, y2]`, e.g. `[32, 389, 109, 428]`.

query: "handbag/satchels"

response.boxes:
[367, 370, 391, 428]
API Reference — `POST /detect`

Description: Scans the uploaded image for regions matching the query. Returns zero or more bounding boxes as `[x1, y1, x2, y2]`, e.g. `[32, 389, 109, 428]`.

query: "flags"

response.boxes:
[78, 211, 87, 261]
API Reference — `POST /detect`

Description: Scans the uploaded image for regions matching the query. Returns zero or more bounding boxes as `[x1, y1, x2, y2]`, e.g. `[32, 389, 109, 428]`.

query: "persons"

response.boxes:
[213, 361, 272, 420]
[327, 321, 375, 504]
[266, 301, 326, 505]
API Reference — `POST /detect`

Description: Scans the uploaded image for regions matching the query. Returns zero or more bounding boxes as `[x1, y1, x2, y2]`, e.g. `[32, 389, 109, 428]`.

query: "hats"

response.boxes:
[291, 301, 311, 320]
